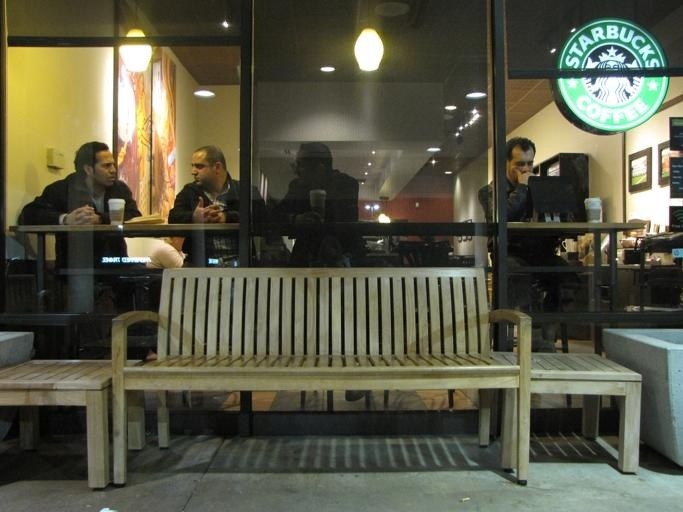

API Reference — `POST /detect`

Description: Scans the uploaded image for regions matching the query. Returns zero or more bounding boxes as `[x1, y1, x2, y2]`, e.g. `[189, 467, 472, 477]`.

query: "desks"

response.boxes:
[7, 219, 648, 415]
[0, 357, 146, 491]
[490, 350, 645, 477]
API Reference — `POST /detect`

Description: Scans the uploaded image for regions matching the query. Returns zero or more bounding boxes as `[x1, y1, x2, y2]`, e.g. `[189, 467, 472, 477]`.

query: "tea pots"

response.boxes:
[561, 239, 574, 251]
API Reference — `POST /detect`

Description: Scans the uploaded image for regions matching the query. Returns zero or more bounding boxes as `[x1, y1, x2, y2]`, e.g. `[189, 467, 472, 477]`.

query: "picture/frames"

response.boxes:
[627, 146, 651, 193]
[657, 139, 670, 186]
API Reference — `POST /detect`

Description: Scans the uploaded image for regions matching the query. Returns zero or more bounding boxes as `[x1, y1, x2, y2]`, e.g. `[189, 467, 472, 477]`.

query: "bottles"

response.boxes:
[577, 233, 605, 259]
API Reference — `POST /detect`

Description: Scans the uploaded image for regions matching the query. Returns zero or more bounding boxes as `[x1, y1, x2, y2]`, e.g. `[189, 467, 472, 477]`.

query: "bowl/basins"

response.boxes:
[620, 240, 635, 248]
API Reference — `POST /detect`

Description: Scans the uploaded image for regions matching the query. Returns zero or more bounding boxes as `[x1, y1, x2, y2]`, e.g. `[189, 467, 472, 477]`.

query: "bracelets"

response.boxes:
[63, 213, 68, 224]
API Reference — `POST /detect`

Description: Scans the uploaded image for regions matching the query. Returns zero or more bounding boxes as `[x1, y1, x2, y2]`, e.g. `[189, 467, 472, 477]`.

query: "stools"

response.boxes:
[506, 272, 574, 407]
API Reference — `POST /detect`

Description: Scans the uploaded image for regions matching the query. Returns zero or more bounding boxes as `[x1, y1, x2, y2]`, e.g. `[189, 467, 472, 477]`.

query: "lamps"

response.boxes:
[117, 0, 153, 73]
[377, 196, 392, 223]
[352, 1, 384, 72]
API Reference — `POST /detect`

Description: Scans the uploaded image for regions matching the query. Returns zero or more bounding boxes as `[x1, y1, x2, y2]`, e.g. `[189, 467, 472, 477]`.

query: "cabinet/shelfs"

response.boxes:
[532, 152, 588, 177]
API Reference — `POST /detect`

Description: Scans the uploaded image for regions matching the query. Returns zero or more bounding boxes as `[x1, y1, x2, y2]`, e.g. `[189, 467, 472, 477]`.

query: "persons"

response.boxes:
[167, 145, 272, 267]
[477, 138, 579, 351]
[264, 142, 369, 401]
[16, 140, 141, 286]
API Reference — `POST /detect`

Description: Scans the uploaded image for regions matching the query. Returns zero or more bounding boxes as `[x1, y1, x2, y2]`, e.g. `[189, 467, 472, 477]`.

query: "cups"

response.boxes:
[107, 198, 125, 225]
[583, 197, 600, 222]
[309, 190, 326, 223]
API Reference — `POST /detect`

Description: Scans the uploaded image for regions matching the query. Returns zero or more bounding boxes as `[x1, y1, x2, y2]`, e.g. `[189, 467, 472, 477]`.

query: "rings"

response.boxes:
[74, 215, 80, 220]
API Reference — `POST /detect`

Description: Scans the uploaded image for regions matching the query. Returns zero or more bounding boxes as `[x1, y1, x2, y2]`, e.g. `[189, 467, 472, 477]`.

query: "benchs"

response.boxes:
[110, 266, 532, 487]
